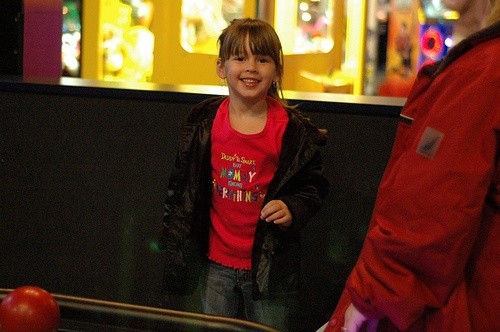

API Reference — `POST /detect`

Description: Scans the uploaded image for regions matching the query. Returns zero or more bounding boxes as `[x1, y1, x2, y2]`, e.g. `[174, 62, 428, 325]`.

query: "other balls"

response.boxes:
[0, 285, 58, 332]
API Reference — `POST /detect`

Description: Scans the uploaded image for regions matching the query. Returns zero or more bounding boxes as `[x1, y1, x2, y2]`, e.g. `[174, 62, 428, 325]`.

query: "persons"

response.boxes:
[159, 18, 330, 332]
[318, 0, 500, 332]
